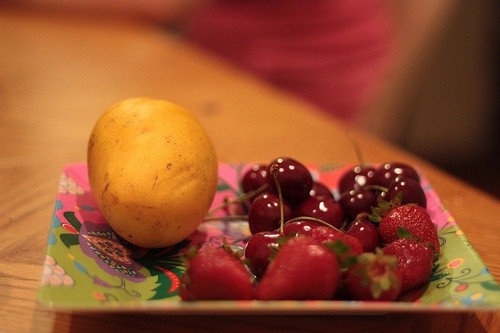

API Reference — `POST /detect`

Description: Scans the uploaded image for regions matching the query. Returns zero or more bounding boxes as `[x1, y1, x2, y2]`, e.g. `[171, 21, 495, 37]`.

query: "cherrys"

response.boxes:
[238, 155, 427, 276]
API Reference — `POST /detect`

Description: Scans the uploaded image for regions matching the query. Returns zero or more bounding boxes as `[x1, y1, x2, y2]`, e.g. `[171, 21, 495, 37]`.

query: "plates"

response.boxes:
[36, 164, 500, 317]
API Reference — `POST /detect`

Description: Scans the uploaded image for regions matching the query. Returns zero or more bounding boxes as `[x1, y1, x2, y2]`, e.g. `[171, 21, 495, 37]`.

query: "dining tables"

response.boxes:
[1, 0, 500, 332]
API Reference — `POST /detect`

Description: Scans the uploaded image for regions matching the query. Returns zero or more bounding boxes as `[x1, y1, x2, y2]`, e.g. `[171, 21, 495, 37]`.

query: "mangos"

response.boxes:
[87, 94, 218, 249]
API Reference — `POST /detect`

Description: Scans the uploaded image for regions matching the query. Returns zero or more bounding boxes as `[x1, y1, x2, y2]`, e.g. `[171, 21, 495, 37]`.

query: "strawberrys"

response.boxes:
[179, 201, 439, 302]
